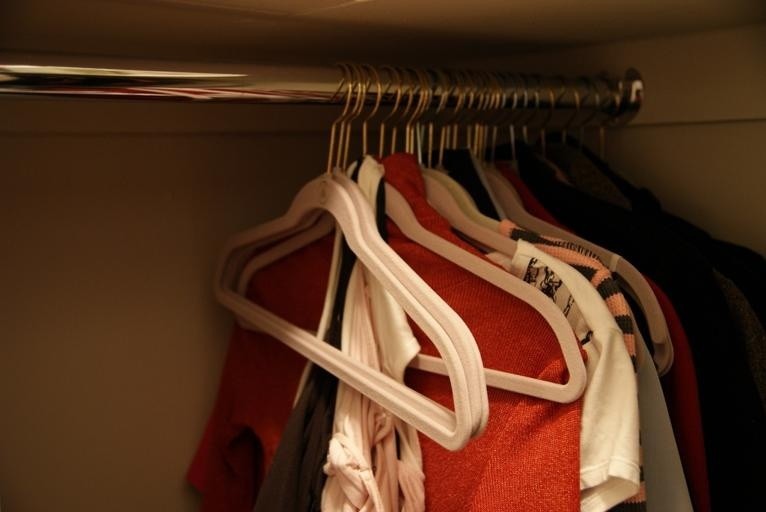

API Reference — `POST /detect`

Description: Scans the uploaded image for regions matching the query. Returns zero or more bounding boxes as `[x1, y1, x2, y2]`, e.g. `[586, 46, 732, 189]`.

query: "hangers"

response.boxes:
[206, 65, 645, 452]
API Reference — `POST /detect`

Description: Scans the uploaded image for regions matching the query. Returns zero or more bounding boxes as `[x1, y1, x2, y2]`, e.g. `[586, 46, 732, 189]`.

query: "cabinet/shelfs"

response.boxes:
[0, 0, 764, 512]
